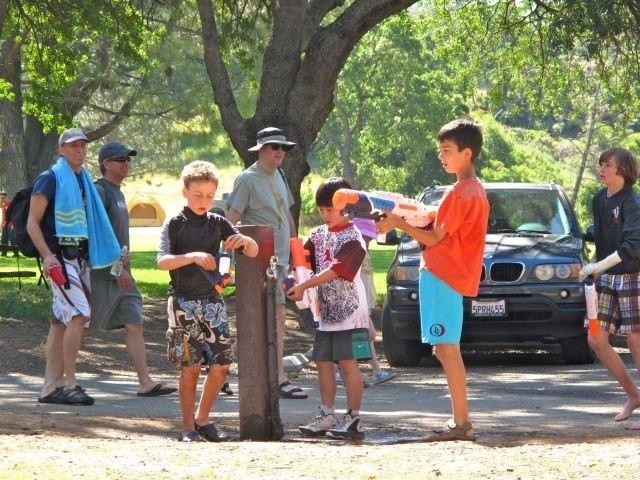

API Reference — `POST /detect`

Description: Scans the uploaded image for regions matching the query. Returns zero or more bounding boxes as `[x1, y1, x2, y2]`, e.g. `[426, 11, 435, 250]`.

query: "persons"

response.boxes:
[157, 161, 259, 443]
[0, 190, 20, 258]
[335, 235, 398, 387]
[27, 128, 95, 405]
[578, 148, 640, 430]
[206, 126, 308, 399]
[373, 118, 490, 441]
[55, 142, 178, 395]
[285, 176, 370, 440]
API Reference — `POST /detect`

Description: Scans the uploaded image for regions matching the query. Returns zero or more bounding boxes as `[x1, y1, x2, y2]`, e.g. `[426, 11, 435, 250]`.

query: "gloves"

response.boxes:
[578, 251, 622, 285]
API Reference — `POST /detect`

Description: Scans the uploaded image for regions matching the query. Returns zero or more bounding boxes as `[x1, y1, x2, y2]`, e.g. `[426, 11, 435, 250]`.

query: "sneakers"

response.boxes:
[325, 413, 365, 440]
[298, 406, 339, 438]
[62, 386, 93, 404]
[373, 370, 396, 385]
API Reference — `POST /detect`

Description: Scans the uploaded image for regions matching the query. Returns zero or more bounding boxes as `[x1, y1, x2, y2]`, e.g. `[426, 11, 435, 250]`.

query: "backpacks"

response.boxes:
[6, 169, 56, 258]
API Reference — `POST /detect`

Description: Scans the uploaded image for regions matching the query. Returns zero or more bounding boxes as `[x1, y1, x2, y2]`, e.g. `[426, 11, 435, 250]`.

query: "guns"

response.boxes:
[194, 241, 236, 299]
[333, 187, 438, 246]
[585, 277, 601, 338]
[285, 237, 320, 332]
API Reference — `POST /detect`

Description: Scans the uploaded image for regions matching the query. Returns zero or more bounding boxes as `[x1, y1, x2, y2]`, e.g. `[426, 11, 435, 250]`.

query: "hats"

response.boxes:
[247, 127, 297, 154]
[98, 143, 137, 162]
[59, 127, 89, 146]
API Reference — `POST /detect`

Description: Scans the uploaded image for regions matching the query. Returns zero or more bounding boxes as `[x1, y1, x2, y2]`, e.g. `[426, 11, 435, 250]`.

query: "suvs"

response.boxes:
[381, 181, 596, 366]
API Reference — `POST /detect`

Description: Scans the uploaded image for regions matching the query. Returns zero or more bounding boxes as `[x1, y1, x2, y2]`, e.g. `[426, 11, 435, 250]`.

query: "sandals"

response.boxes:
[195, 421, 228, 441]
[419, 418, 477, 443]
[177, 430, 209, 442]
[278, 380, 308, 400]
[206, 366, 233, 395]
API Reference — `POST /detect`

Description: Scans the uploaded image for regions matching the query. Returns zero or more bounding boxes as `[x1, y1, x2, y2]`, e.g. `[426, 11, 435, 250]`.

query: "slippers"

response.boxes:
[136, 384, 177, 397]
[38, 390, 66, 404]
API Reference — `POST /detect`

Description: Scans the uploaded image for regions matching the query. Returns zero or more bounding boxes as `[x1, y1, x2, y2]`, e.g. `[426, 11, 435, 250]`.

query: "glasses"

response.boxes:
[266, 143, 287, 152]
[108, 157, 131, 162]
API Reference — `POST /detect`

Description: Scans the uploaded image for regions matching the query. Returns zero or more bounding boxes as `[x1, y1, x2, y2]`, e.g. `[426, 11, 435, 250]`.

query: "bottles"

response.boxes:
[109, 245, 128, 277]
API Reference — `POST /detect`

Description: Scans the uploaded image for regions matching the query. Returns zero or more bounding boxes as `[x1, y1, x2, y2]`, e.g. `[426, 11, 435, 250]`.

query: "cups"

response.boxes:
[48, 265, 66, 286]
